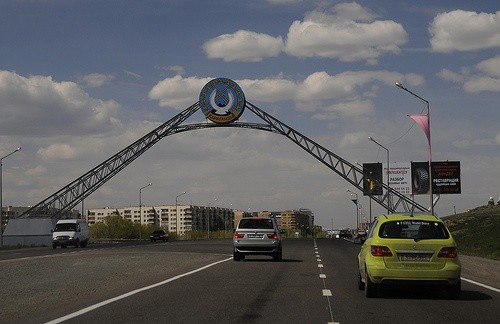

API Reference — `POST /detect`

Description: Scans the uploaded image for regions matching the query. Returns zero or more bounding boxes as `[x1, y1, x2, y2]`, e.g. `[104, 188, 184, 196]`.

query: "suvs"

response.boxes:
[232, 216, 286, 262]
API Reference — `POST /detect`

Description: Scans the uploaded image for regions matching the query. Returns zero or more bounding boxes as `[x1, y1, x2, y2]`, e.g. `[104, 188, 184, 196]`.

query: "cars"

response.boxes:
[356, 212, 462, 300]
[339, 230, 352, 238]
[149, 230, 169, 243]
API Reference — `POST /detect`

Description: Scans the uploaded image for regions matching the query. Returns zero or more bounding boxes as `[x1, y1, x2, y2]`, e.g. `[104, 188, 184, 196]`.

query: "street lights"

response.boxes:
[224, 203, 232, 239]
[346, 189, 358, 234]
[207, 198, 218, 241]
[367, 136, 390, 216]
[139, 182, 152, 242]
[395, 81, 434, 216]
[175, 191, 186, 241]
[0, 147, 22, 250]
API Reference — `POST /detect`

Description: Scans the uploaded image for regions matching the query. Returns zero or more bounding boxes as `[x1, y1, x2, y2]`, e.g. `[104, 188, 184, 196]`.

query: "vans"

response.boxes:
[50, 218, 90, 250]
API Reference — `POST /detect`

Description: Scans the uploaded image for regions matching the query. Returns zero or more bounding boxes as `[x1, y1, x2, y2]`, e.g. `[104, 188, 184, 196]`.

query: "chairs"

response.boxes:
[384, 223, 402, 239]
[416, 224, 434, 239]
